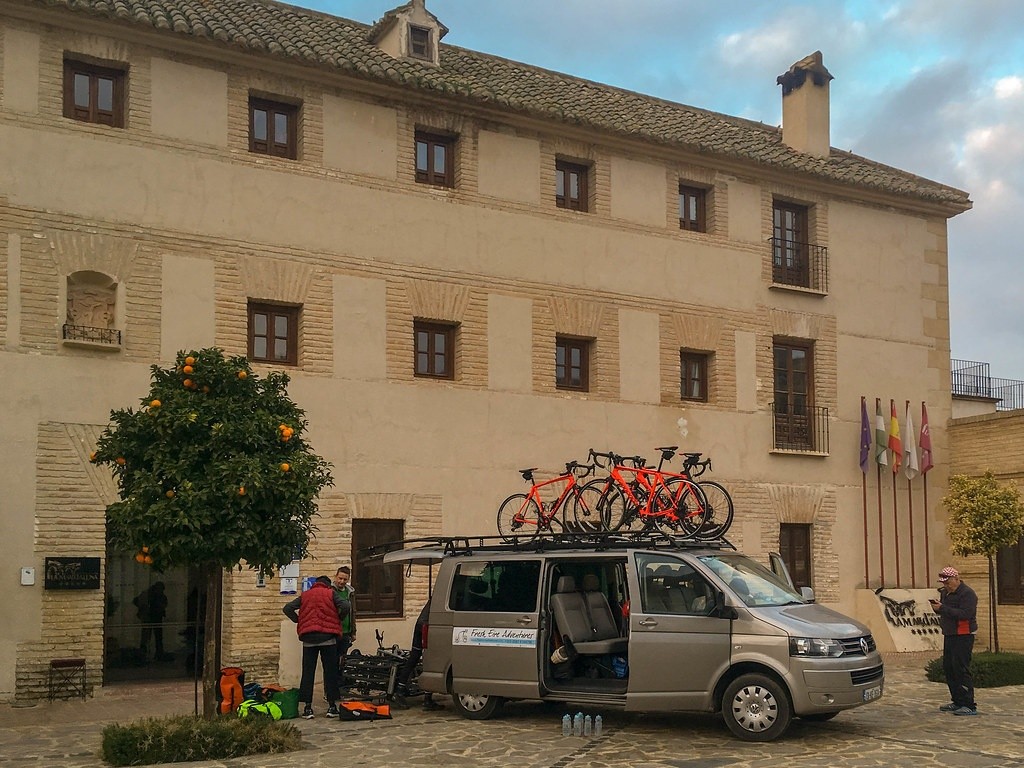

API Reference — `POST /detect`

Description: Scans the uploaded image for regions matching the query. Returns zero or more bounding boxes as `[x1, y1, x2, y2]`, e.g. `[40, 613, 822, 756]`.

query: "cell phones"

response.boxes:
[929, 599, 937, 604]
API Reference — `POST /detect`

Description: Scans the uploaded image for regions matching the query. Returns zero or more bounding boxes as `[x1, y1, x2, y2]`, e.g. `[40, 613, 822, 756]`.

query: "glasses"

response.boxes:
[939, 574, 953, 578]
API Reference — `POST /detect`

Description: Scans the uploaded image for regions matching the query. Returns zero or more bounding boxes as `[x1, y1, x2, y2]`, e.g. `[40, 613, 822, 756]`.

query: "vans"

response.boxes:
[382, 550, 886, 743]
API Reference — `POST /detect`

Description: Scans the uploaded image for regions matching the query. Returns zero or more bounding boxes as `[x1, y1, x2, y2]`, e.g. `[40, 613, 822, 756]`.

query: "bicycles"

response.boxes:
[335, 629, 426, 703]
[574, 445, 735, 541]
[495, 461, 612, 547]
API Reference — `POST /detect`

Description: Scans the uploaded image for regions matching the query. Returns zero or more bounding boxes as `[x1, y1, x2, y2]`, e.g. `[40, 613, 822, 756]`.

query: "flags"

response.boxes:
[888, 403, 903, 475]
[919, 405, 934, 476]
[876, 400, 888, 471]
[860, 399, 872, 473]
[904, 404, 918, 479]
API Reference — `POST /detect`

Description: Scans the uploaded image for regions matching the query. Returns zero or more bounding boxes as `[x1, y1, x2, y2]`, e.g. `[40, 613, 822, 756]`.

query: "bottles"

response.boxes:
[561, 712, 603, 737]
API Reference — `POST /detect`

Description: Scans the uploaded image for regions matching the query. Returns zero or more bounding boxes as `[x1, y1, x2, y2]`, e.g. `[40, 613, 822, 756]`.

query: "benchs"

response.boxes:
[551, 574, 630, 677]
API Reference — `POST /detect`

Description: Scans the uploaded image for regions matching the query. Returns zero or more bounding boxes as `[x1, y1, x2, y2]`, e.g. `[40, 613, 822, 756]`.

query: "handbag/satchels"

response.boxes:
[237, 698, 283, 722]
[262, 684, 283, 699]
[339, 701, 393, 721]
[271, 688, 301, 718]
[243, 683, 262, 699]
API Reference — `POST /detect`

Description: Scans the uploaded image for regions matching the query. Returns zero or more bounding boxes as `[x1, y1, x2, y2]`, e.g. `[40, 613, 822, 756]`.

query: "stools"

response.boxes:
[49, 657, 87, 703]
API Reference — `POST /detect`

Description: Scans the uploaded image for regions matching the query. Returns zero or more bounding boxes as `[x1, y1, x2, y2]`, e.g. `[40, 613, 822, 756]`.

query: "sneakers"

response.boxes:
[953, 706, 977, 715]
[302, 708, 315, 719]
[326, 708, 339, 717]
[940, 703, 959, 711]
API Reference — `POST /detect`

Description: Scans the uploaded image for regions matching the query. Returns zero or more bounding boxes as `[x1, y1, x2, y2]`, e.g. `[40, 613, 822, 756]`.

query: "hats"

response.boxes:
[937, 568, 958, 582]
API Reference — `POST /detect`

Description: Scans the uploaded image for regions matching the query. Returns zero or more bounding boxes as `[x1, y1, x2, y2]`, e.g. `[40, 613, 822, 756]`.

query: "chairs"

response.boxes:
[640, 565, 713, 614]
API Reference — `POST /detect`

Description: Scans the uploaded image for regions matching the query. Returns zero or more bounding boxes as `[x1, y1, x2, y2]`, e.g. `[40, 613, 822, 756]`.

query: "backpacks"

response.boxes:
[215, 667, 246, 715]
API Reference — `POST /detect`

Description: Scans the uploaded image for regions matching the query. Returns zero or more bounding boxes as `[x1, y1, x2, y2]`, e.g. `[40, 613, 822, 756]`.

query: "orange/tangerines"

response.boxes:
[90, 357, 293, 563]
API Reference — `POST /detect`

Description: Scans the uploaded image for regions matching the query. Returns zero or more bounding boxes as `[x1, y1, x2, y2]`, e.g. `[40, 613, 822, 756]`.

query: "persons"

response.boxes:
[930, 567, 977, 715]
[282, 576, 349, 719]
[133, 581, 167, 660]
[729, 579, 756, 605]
[331, 566, 357, 655]
